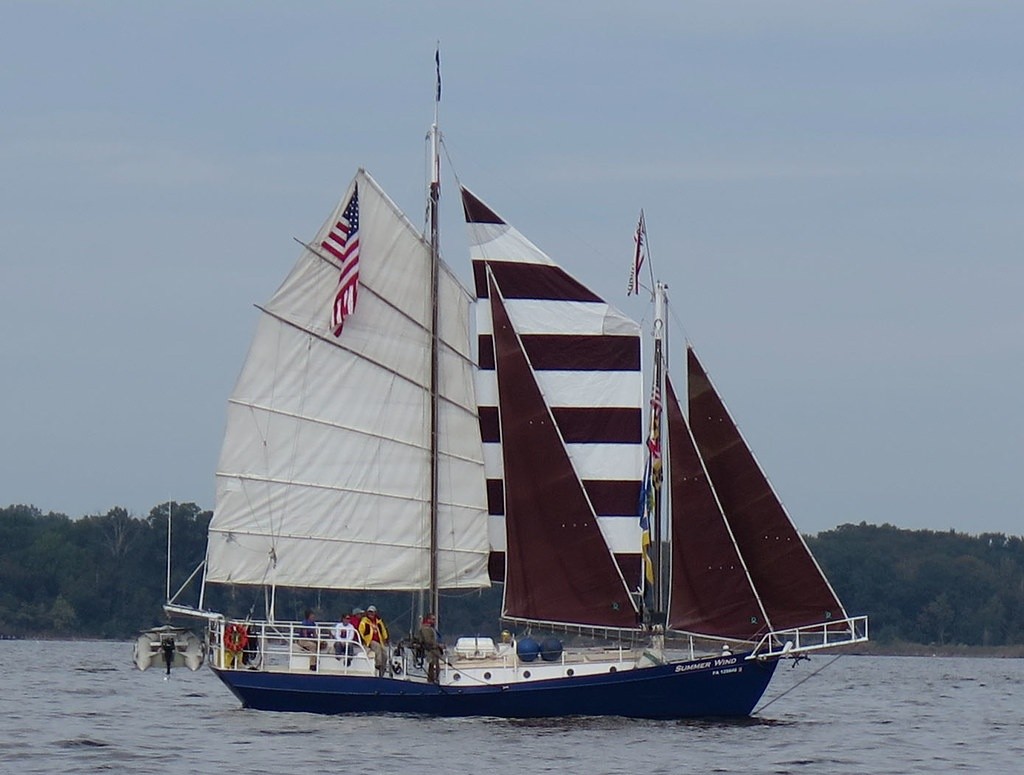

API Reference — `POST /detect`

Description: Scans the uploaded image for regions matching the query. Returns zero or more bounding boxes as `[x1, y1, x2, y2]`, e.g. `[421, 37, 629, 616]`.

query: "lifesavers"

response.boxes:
[224, 624, 248, 652]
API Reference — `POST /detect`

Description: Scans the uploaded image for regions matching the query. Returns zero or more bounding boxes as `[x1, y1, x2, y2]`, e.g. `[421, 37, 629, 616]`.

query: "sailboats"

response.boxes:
[162, 40, 871, 721]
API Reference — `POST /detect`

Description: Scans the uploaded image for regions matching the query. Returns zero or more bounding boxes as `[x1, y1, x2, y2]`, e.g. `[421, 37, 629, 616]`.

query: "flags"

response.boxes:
[627, 219, 647, 296]
[322, 180, 360, 339]
[637, 351, 661, 599]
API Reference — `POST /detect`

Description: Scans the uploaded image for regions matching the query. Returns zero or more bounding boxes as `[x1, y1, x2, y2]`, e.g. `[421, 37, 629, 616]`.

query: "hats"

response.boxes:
[367, 605, 377, 612]
[352, 608, 365, 615]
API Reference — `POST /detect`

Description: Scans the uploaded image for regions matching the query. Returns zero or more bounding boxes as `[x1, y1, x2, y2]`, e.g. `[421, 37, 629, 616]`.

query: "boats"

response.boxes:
[131, 623, 205, 672]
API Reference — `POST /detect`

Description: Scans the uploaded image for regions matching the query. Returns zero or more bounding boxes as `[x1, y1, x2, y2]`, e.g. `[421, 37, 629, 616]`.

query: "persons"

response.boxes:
[299, 605, 444, 682]
